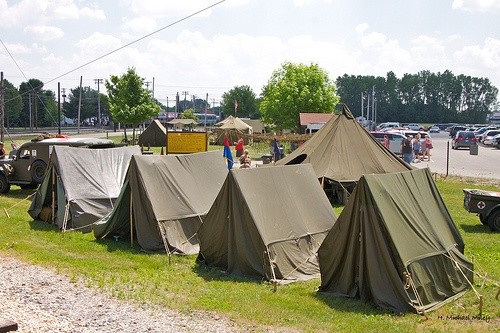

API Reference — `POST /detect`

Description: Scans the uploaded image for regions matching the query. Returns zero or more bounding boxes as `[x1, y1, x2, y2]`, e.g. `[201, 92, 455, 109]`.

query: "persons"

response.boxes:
[239, 149, 251, 167]
[384, 135, 389, 148]
[280, 144, 284, 154]
[404, 133, 431, 163]
[234, 134, 244, 157]
[11, 143, 16, 150]
[0, 142, 6, 159]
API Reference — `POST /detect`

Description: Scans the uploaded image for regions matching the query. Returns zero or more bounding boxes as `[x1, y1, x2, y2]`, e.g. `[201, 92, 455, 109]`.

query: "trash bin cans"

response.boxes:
[261, 155, 273, 165]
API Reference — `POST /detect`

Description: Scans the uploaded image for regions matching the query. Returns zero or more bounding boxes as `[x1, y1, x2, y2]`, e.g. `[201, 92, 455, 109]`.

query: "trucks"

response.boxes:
[463, 189, 500, 233]
[0, 137, 129, 195]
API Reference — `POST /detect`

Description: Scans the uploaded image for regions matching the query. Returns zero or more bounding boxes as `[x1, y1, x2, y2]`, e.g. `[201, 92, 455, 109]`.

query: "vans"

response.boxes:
[368, 122, 433, 155]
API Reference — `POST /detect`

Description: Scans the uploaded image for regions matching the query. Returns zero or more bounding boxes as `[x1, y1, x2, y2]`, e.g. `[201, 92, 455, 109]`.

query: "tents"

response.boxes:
[91, 149, 230, 255]
[139, 119, 167, 147]
[195, 164, 337, 284]
[212, 114, 253, 145]
[256, 103, 418, 208]
[316, 168, 475, 314]
[27, 145, 143, 229]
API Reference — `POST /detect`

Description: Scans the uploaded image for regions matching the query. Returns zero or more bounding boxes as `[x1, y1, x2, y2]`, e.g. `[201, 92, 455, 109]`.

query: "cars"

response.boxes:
[452, 130, 478, 150]
[448, 121, 500, 150]
[430, 126, 440, 133]
